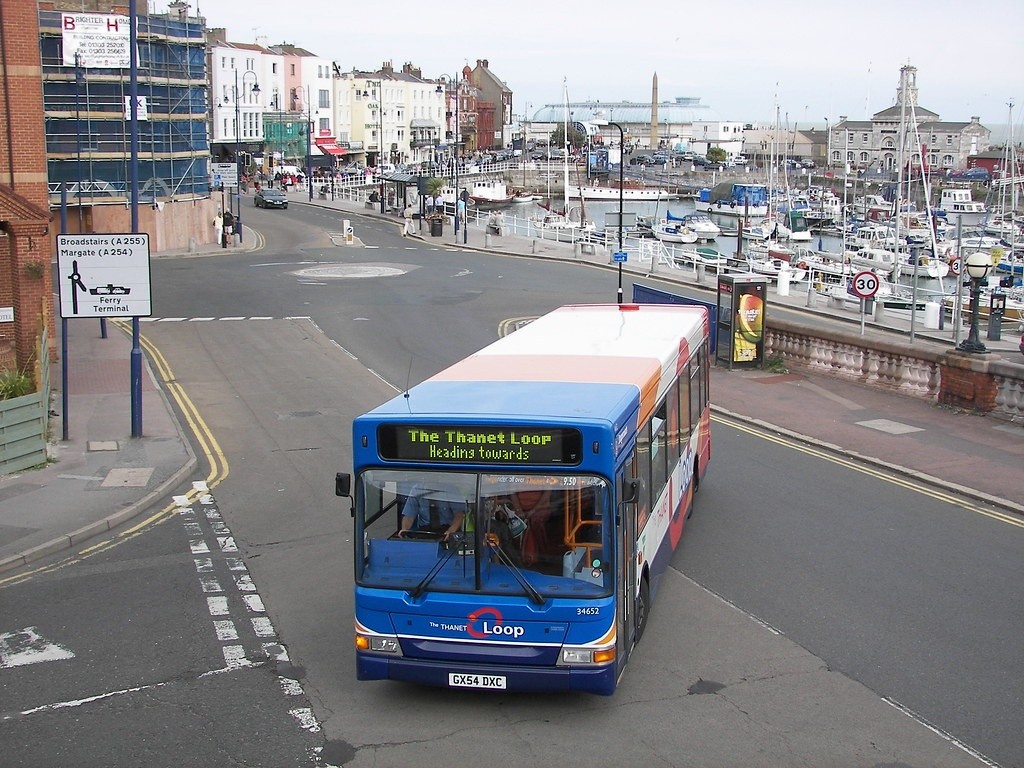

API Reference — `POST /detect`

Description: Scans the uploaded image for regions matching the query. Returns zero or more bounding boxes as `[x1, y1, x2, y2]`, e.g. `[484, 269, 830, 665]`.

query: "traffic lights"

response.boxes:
[269, 154, 273, 168]
[241, 152, 251, 167]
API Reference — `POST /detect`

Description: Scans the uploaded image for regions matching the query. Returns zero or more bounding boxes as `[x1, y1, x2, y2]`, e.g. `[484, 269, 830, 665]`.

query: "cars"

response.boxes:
[780, 159, 801, 170]
[476, 143, 536, 166]
[253, 188, 288, 210]
[375, 164, 395, 175]
[531, 149, 544, 160]
[630, 154, 654, 166]
[800, 158, 814, 168]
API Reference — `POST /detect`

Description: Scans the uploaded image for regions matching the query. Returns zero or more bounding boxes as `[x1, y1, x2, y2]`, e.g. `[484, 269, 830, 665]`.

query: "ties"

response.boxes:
[426, 491, 440, 530]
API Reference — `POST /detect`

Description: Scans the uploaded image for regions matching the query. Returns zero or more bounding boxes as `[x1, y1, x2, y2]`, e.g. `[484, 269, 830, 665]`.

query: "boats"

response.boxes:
[635, 183, 769, 269]
[428, 181, 543, 221]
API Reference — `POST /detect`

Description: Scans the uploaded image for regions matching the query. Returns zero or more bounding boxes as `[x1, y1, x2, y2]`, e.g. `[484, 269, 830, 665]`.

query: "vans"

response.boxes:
[276, 166, 306, 182]
[421, 161, 439, 173]
[653, 155, 668, 165]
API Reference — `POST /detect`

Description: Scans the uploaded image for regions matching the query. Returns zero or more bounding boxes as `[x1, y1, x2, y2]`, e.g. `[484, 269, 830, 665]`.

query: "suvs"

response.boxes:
[964, 167, 988, 179]
[317, 166, 339, 177]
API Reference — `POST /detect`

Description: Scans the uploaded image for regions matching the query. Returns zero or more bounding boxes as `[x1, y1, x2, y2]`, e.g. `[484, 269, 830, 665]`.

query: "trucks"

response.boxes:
[734, 156, 748, 165]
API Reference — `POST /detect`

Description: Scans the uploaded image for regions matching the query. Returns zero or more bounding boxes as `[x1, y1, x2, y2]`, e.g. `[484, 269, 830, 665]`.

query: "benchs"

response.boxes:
[286, 186, 295, 192]
[365, 202, 381, 210]
[486, 225, 509, 236]
[575, 241, 604, 255]
[391, 207, 403, 217]
[318, 192, 332, 200]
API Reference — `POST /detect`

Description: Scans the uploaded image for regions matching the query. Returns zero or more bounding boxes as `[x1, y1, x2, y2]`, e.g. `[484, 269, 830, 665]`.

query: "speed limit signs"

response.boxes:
[853, 271, 880, 299]
[952, 257, 965, 273]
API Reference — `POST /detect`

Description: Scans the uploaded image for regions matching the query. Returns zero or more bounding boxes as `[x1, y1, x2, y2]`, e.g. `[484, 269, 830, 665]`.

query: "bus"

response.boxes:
[334, 304, 711, 695]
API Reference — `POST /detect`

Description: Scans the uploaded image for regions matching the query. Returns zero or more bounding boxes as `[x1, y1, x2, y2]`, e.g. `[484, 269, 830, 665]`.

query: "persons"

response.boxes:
[388, 187, 395, 206]
[398, 473, 466, 543]
[489, 210, 504, 234]
[457, 198, 465, 224]
[509, 485, 552, 566]
[370, 191, 380, 202]
[241, 167, 303, 193]
[402, 204, 416, 237]
[224, 208, 234, 244]
[336, 167, 371, 182]
[213, 211, 223, 245]
[427, 196, 433, 215]
[435, 195, 444, 215]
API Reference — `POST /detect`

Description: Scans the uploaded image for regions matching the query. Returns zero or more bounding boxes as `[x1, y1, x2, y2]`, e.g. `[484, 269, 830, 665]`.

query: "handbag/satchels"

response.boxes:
[515, 518, 539, 567]
[502, 504, 527, 539]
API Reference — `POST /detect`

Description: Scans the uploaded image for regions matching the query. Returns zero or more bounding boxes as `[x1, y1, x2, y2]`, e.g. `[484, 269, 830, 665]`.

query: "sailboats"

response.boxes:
[530, 75, 596, 242]
[743, 57, 1024, 330]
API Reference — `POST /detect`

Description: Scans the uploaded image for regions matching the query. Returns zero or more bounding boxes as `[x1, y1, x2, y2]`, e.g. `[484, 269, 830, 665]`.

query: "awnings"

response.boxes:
[304, 145, 324, 156]
[317, 143, 346, 155]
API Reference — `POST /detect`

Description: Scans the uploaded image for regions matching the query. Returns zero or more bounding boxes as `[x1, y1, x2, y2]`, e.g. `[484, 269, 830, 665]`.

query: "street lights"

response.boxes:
[234, 69, 261, 243]
[363, 75, 386, 213]
[955, 253, 992, 353]
[590, 117, 623, 305]
[435, 73, 459, 230]
[293, 83, 314, 200]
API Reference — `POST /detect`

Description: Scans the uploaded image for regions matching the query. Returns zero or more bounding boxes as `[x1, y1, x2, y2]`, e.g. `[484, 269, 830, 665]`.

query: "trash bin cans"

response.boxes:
[431, 217, 443, 238]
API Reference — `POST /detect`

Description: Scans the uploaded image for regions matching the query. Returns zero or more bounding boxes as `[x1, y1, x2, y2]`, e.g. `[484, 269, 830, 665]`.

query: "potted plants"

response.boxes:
[424, 177, 449, 232]
